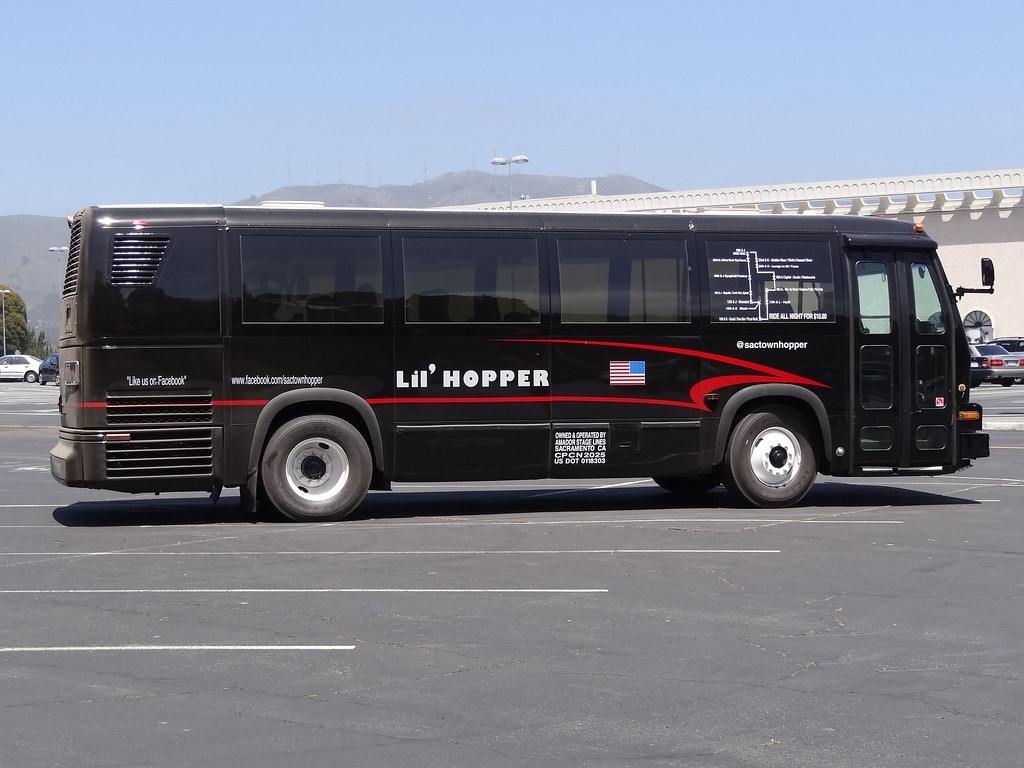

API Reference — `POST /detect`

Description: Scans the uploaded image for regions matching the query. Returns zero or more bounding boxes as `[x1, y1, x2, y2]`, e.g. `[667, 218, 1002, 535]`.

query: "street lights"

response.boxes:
[490, 155, 529, 213]
[0, 289, 11, 356]
[48, 247, 69, 301]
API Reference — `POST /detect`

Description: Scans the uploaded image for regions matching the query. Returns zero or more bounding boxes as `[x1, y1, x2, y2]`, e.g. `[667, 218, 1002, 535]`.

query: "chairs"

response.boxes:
[305, 289, 534, 323]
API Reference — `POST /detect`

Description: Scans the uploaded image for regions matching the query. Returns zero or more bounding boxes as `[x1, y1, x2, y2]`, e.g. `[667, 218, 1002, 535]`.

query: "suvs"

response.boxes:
[986, 336, 1024, 384]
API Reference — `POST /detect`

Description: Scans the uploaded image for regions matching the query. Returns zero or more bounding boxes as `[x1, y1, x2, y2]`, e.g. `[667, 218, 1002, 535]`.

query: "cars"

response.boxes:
[0, 354, 45, 384]
[968, 343, 1024, 387]
[39, 353, 60, 386]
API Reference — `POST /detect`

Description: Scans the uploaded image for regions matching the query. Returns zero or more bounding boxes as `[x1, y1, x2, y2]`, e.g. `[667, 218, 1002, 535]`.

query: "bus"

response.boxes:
[43, 204, 997, 523]
